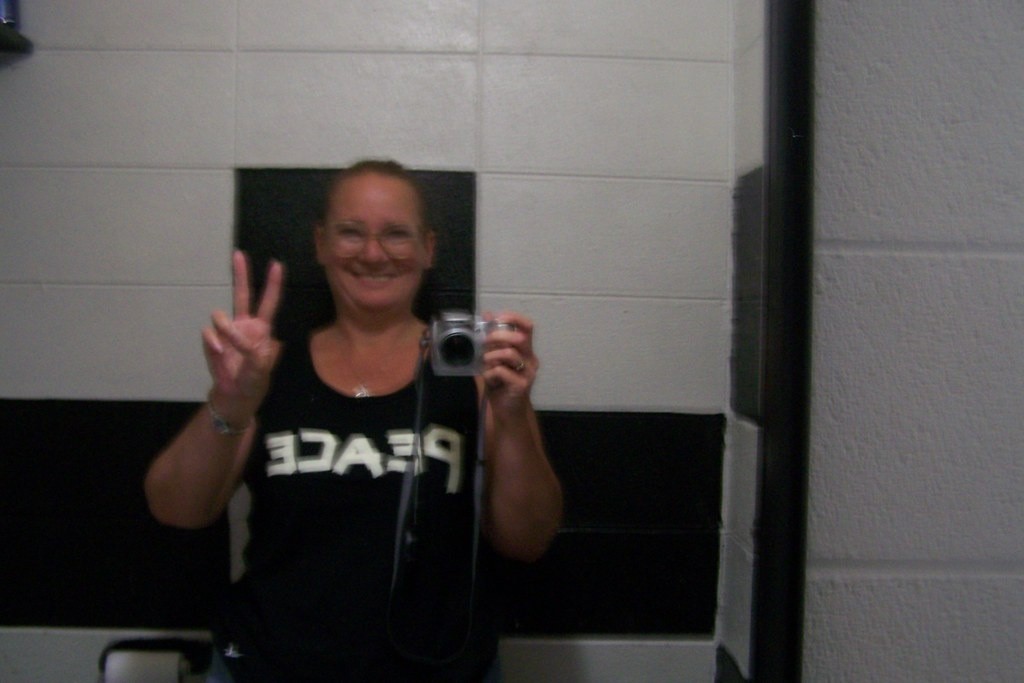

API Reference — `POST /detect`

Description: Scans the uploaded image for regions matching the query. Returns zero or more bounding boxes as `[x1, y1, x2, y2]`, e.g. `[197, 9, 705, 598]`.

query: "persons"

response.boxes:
[145, 160, 564, 683]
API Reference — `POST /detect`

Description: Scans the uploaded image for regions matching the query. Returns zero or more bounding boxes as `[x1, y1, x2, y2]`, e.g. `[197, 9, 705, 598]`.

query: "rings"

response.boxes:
[516, 362, 524, 371]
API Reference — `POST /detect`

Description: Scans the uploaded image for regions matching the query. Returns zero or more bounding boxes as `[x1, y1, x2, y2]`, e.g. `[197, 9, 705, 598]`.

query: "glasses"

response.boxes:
[321, 219, 427, 260]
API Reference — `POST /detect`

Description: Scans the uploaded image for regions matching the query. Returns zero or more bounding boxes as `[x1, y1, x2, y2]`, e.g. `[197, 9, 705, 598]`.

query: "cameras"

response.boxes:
[429, 311, 514, 376]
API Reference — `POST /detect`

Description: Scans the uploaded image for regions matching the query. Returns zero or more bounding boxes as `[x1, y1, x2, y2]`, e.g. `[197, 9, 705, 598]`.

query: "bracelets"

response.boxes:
[207, 390, 250, 435]
